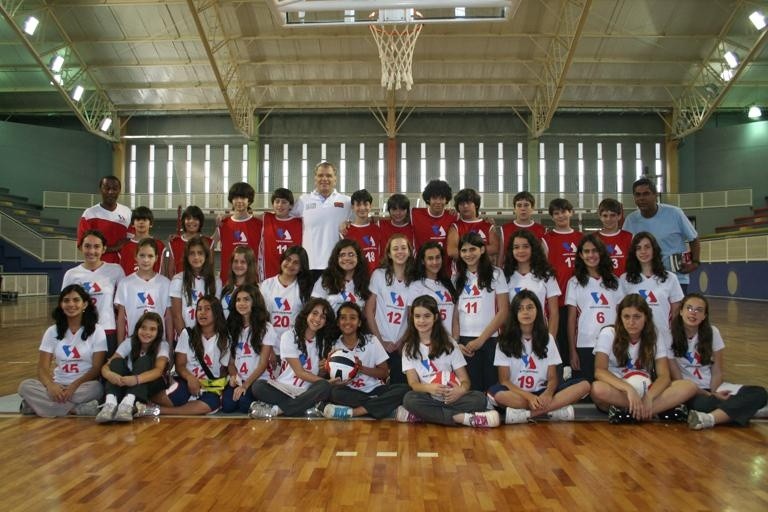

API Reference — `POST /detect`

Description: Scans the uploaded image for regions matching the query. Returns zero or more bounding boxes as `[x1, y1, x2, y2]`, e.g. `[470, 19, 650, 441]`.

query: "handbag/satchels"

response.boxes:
[198, 376, 228, 396]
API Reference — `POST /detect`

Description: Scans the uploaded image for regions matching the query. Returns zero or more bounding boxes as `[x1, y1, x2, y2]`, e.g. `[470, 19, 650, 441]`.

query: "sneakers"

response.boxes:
[655, 403, 691, 422]
[247, 400, 277, 419]
[133, 400, 161, 418]
[504, 406, 533, 425]
[323, 403, 355, 420]
[396, 405, 424, 424]
[687, 409, 713, 430]
[94, 402, 133, 423]
[469, 409, 501, 428]
[608, 405, 638, 425]
[304, 400, 326, 418]
[75, 399, 101, 416]
[19, 400, 36, 414]
[547, 404, 575, 422]
[753, 404, 768, 419]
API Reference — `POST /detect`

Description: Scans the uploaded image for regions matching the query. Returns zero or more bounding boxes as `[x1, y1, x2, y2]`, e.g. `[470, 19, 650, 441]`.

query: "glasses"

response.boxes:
[686, 306, 706, 313]
[336, 253, 358, 257]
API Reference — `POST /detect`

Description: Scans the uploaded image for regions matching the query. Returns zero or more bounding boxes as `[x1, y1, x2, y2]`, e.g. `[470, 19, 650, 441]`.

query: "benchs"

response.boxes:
[0, 187, 80, 243]
[692, 193, 766, 237]
